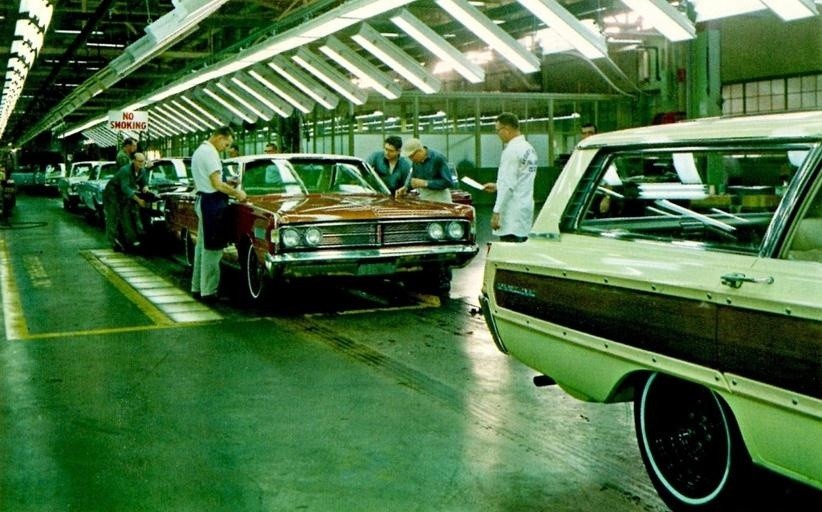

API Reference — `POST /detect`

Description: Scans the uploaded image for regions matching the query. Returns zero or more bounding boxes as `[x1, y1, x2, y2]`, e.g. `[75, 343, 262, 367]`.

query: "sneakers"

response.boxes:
[112, 237, 129, 252]
[193, 293, 230, 302]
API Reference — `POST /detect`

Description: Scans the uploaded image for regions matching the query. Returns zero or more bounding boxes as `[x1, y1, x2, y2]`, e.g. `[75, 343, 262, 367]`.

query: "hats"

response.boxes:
[405, 138, 423, 157]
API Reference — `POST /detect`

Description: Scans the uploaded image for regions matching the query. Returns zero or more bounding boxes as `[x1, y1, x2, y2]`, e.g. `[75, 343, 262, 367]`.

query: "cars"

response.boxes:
[160, 153, 480, 306]
[132, 156, 195, 242]
[0, 150, 119, 224]
[481, 110, 822, 512]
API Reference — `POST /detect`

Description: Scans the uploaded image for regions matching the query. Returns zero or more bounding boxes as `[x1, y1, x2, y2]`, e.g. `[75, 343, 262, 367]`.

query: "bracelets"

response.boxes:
[424, 180, 428, 189]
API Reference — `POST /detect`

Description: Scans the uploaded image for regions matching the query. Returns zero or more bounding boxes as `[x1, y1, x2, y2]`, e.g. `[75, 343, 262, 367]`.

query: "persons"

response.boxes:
[580, 123, 621, 187]
[371, 135, 409, 193]
[265, 144, 281, 184]
[482, 111, 537, 242]
[394, 139, 454, 203]
[102, 152, 160, 251]
[229, 142, 240, 157]
[116, 137, 138, 240]
[189, 125, 249, 300]
